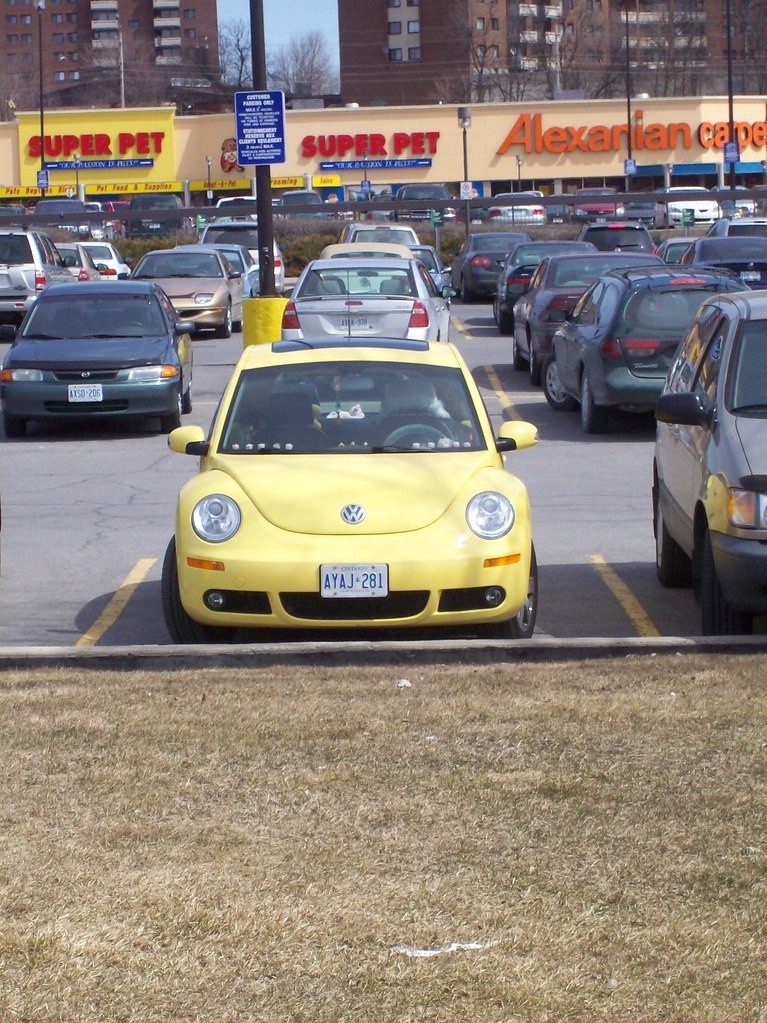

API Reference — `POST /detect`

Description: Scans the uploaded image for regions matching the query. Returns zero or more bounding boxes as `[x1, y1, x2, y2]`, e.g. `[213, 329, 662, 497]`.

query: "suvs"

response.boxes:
[704, 215, 767, 237]
[337, 224, 422, 258]
[0, 224, 75, 314]
[578, 219, 659, 254]
[199, 220, 285, 294]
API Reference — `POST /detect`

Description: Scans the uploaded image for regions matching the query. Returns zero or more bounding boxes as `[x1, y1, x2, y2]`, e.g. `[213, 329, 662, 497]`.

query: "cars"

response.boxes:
[162, 334, 541, 646]
[492, 240, 611, 335]
[655, 236, 701, 267]
[165, 243, 261, 301]
[72, 241, 133, 280]
[538, 265, 752, 437]
[450, 232, 533, 303]
[117, 248, 244, 338]
[280, 257, 457, 344]
[318, 243, 452, 295]
[54, 241, 109, 282]
[651, 289, 767, 638]
[679, 235, 767, 291]
[507, 251, 666, 387]
[0, 183, 767, 242]
[0, 280, 195, 439]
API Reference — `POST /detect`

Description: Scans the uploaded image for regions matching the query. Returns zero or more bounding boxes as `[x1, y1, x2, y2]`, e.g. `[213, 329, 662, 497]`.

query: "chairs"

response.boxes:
[54, 309, 90, 334]
[377, 377, 449, 438]
[263, 383, 325, 439]
[315, 276, 346, 295]
[155, 262, 171, 277]
[378, 278, 409, 295]
[196, 260, 217, 276]
[123, 307, 153, 330]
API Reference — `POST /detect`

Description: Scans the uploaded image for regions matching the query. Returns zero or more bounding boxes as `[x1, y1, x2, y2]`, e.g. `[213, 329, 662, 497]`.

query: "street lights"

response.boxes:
[33, 1, 47, 201]
[456, 105, 471, 237]
